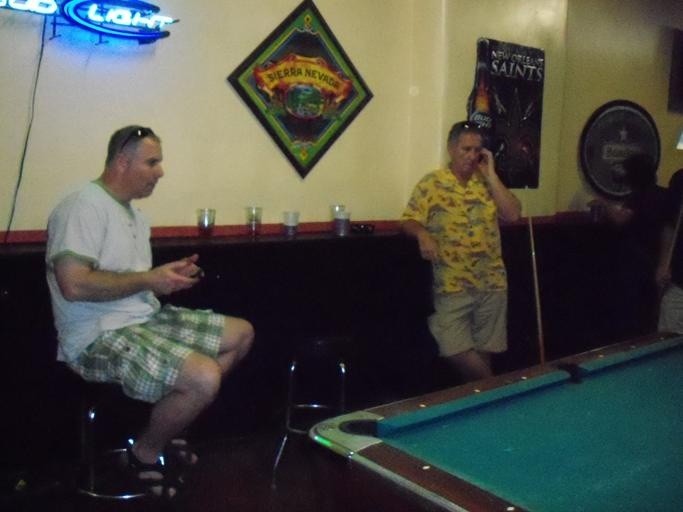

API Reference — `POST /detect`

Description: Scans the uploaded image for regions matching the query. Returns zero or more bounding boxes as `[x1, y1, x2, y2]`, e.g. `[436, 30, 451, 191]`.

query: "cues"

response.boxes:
[650, 204, 683, 334]
[525, 185, 547, 364]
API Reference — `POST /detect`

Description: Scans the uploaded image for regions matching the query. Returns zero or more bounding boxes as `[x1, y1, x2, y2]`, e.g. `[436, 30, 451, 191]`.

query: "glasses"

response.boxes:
[454, 123, 485, 132]
[350, 223, 375, 233]
[119, 126, 152, 154]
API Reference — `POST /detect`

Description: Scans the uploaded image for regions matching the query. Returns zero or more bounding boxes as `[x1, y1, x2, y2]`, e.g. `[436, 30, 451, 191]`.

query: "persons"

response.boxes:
[653, 129, 682, 335]
[586, 152, 666, 342]
[397, 115, 523, 384]
[41, 122, 256, 500]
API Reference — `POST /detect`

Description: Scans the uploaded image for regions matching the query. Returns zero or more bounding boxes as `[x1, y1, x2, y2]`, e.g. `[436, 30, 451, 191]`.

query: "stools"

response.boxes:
[54, 362, 147, 502]
[269, 335, 354, 492]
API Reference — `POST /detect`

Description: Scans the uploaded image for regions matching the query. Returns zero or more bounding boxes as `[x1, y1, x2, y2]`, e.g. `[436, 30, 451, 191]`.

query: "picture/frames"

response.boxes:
[226, 0, 373, 180]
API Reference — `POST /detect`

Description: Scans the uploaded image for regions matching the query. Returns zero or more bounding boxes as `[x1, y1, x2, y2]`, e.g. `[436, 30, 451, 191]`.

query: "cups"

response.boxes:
[282, 210, 300, 238]
[328, 201, 351, 238]
[195, 208, 216, 235]
[243, 200, 263, 238]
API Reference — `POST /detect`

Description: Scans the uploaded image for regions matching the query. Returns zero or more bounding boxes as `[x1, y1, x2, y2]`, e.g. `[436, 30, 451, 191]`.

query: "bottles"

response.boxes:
[466, 38, 496, 136]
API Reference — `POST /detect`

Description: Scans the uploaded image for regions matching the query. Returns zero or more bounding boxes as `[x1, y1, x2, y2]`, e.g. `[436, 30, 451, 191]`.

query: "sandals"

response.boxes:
[165, 434, 199, 468]
[113, 442, 185, 503]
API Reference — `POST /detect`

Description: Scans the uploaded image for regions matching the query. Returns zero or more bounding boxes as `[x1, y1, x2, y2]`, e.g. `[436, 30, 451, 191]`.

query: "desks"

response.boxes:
[305, 332, 682, 512]
